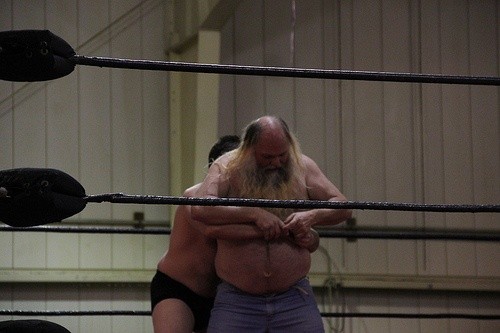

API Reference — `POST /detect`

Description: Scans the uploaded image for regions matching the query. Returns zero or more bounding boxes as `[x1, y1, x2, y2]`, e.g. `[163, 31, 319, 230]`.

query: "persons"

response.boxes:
[150, 134, 321, 333]
[189, 116, 353, 333]
